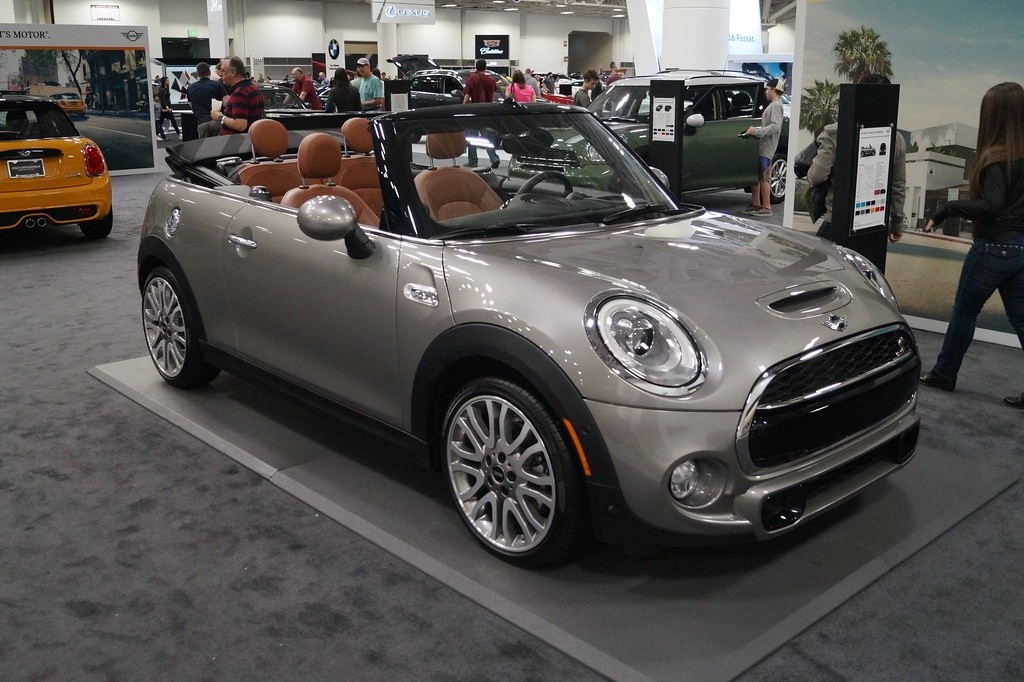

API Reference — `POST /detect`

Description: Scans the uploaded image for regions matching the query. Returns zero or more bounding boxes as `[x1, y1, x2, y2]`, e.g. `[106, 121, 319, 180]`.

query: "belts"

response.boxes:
[974, 241, 1024, 252]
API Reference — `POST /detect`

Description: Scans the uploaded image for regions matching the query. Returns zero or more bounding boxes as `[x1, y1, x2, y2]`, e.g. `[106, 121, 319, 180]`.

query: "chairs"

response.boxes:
[692, 94, 714, 121]
[333, 117, 384, 218]
[729, 93, 753, 117]
[6, 111, 29, 134]
[413, 129, 504, 223]
[230, 118, 302, 203]
[281, 133, 381, 228]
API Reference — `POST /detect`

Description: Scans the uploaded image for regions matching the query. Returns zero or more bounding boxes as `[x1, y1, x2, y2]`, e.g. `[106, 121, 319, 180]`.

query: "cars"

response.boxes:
[0, 89, 114, 239]
[506, 67, 790, 206]
[251, 53, 637, 118]
[50, 93, 86, 118]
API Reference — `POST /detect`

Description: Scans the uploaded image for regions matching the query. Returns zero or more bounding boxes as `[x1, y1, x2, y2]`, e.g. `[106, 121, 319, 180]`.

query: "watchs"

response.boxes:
[221, 116, 227, 125]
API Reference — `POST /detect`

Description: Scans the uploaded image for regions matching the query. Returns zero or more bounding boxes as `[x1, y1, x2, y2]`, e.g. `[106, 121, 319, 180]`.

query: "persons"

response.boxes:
[463, 59, 500, 169]
[284, 75, 289, 81]
[371, 68, 391, 98]
[187, 56, 267, 138]
[247, 71, 272, 84]
[807, 73, 906, 276]
[153, 76, 182, 141]
[574, 69, 600, 107]
[736, 78, 784, 216]
[525, 68, 560, 99]
[350, 58, 384, 112]
[918, 81, 1024, 408]
[283, 67, 323, 110]
[598, 68, 625, 91]
[505, 70, 536, 103]
[325, 67, 362, 113]
[319, 72, 330, 86]
[611, 62, 618, 68]
[306, 72, 312, 79]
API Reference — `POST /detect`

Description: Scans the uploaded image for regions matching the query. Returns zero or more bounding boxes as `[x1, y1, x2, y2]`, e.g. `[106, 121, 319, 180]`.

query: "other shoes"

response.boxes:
[491, 159, 500, 168]
[748, 206, 773, 217]
[157, 135, 163, 141]
[463, 162, 477, 167]
[178, 133, 183, 140]
[919, 369, 957, 392]
[1004, 392, 1024, 410]
[164, 137, 171, 142]
[737, 204, 760, 213]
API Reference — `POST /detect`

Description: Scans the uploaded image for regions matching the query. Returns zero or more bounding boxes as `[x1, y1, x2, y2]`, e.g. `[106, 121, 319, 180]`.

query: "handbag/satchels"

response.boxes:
[805, 166, 833, 223]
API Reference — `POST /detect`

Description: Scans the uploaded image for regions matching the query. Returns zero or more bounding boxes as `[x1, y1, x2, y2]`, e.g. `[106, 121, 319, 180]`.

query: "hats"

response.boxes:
[768, 78, 787, 94]
[357, 58, 370, 66]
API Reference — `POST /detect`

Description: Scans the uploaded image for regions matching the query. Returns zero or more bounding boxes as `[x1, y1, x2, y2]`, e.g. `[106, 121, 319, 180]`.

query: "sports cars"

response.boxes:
[135, 95, 923, 572]
